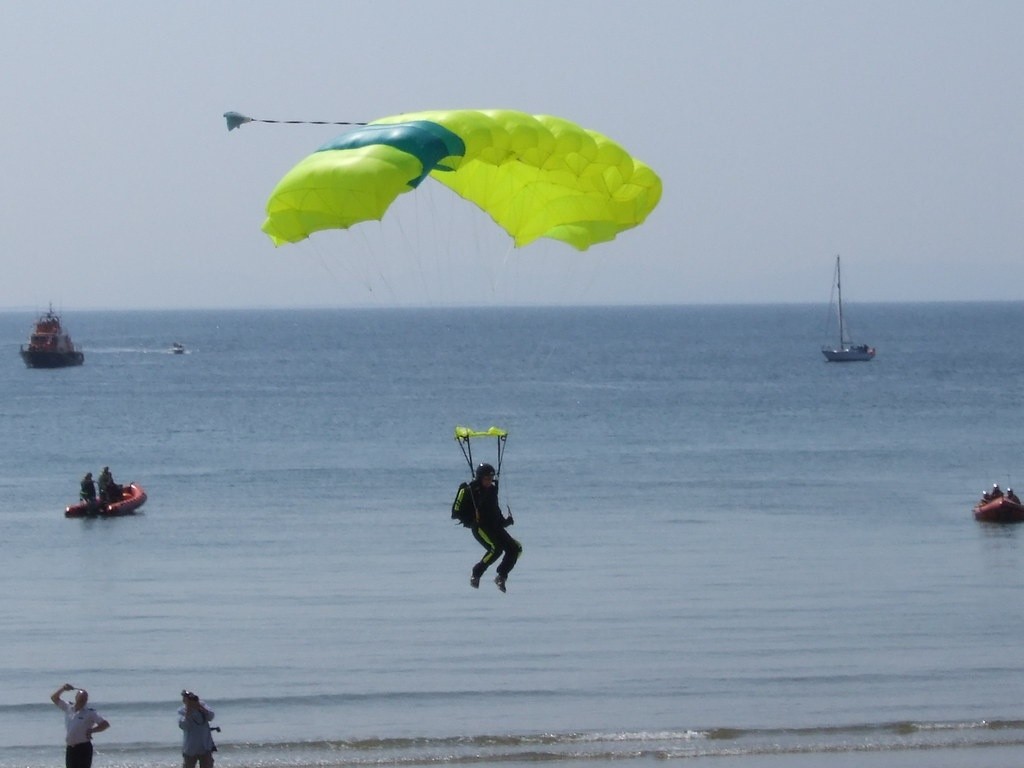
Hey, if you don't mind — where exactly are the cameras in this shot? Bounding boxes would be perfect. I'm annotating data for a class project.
[187,692,198,701]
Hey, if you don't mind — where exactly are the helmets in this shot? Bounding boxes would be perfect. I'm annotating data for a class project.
[476,463,495,480]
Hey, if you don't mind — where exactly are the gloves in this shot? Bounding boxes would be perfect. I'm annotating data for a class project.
[506,516,514,526]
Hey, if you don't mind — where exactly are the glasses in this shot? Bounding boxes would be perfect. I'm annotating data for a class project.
[484,477,494,480]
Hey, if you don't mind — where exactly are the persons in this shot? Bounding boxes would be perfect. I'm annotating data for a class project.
[51,684,109,768]
[468,463,521,593]
[982,484,1021,505]
[80,466,114,502]
[848,343,869,352]
[176,692,217,768]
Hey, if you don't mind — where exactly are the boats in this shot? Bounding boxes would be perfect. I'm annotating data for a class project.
[64,481,146,518]
[974,495,1024,522]
[21,301,85,369]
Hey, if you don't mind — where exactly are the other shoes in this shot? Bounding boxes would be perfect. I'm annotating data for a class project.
[470,572,479,588]
[494,575,506,592]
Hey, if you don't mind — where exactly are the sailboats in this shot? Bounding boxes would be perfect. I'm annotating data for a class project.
[819,251,877,362]
